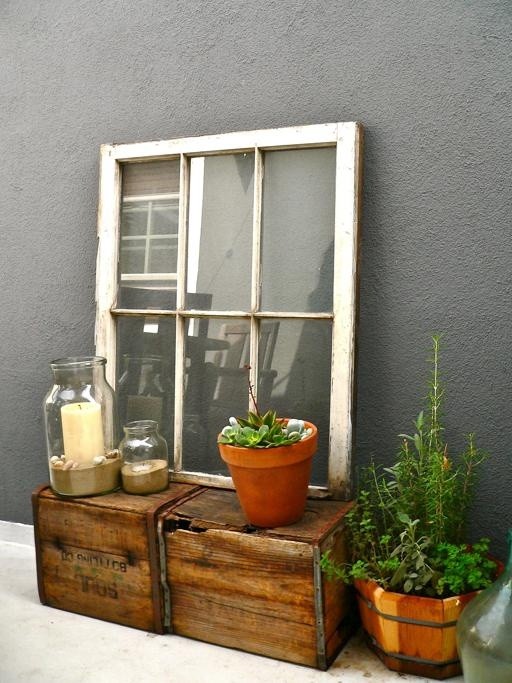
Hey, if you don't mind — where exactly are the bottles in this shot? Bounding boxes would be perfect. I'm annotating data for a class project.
[117,420,170,496]
[42,354,123,498]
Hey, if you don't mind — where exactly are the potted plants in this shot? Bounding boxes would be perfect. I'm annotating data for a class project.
[215,376,319,529]
[317,327,504,679]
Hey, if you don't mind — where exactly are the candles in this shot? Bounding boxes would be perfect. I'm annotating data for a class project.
[60,402,105,465]
